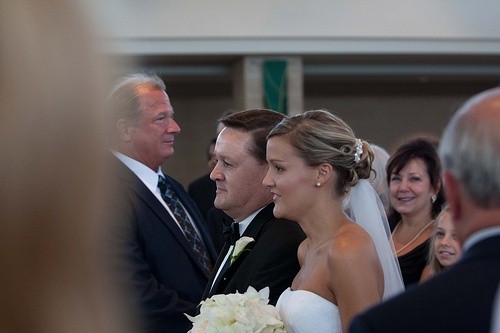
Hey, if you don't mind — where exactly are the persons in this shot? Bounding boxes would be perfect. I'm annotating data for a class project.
[0,0,500,333]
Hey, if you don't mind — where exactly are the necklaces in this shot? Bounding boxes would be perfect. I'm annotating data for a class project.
[391,219,435,253]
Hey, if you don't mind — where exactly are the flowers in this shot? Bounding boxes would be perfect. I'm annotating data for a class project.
[183,286,293,333]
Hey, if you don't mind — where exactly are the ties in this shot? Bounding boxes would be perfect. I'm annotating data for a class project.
[157,175,212,279]
